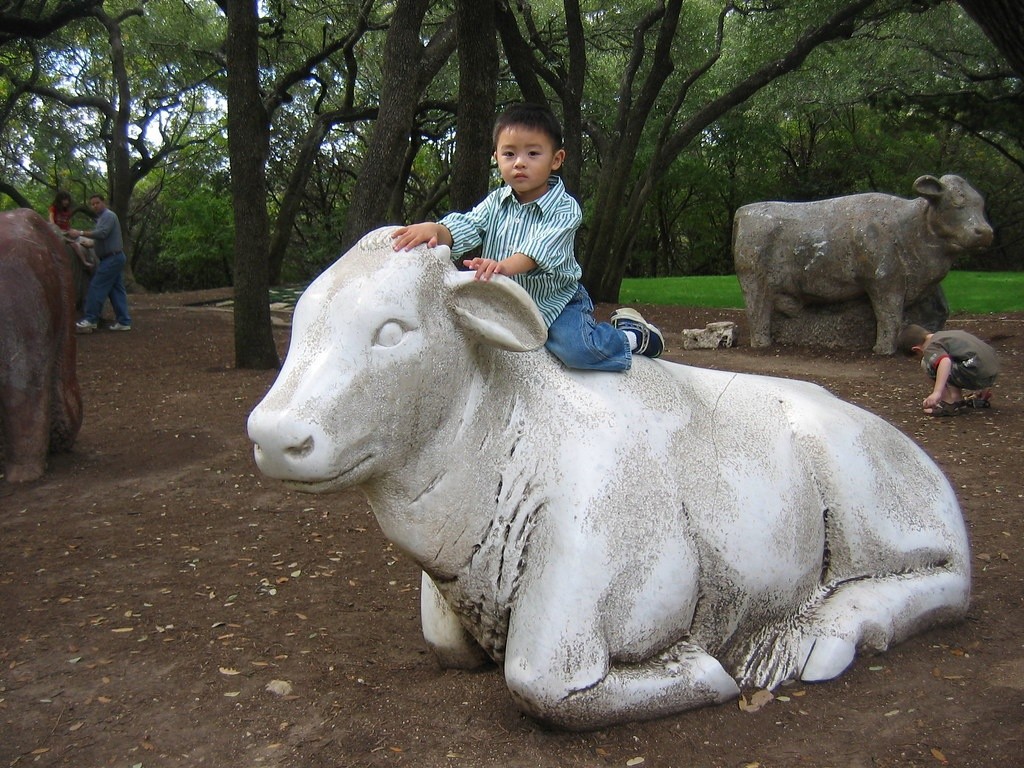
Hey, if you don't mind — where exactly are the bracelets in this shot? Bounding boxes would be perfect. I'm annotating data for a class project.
[80,230,84,236]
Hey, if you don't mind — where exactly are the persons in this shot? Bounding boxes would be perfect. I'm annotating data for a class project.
[899,324,999,417]
[67,194,132,330]
[49,191,95,267]
[391,102,666,371]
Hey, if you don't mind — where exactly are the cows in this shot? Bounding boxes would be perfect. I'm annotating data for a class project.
[0,209,101,491]
[246,224,971,731]
[733,174,994,357]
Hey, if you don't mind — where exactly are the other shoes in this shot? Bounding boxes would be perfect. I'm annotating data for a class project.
[84,262,95,269]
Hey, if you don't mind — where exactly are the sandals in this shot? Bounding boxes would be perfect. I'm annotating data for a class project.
[924,399,970,417]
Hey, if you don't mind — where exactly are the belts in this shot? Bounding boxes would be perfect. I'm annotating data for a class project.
[99,251,123,262]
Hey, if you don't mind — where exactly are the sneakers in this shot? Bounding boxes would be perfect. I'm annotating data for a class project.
[75,320,98,329]
[109,322,131,331]
[610,308,665,360]
